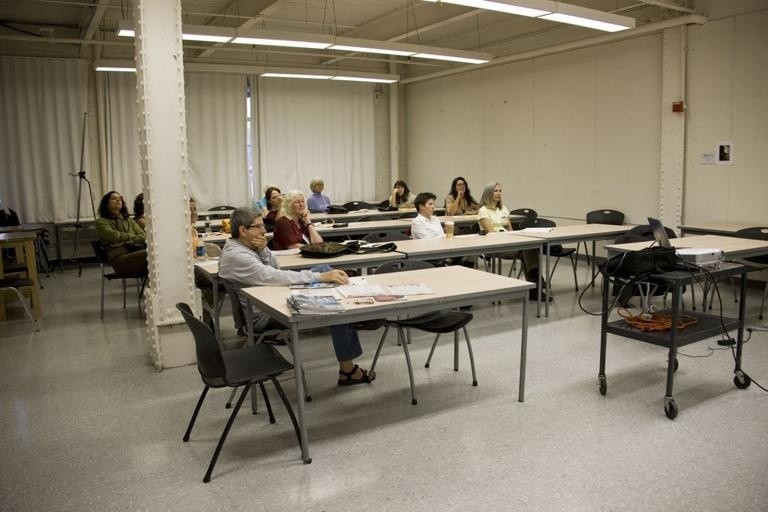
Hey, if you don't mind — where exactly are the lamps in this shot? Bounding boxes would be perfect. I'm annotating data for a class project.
[424,0,636,34]
[94,20,495,84]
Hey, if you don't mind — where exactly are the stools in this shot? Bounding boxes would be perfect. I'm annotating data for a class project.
[0,278,40,333]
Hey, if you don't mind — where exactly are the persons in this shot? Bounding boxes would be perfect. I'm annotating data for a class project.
[130,193,146,231]
[477,182,553,304]
[307,177,333,211]
[387,179,417,208]
[275,190,324,249]
[266,186,280,220]
[409,191,446,244]
[98,190,148,275]
[442,177,483,214]
[189,198,222,292]
[217,205,377,386]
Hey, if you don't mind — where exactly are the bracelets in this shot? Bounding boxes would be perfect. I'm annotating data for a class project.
[304,223,314,228]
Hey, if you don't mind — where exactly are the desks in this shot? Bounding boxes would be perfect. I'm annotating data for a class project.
[240,264,537,466]
[190,198,637,316]
[0,231,41,320]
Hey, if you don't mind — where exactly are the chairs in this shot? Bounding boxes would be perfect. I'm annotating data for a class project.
[91,240,147,319]
[174,302,304,482]
[585,207,767,320]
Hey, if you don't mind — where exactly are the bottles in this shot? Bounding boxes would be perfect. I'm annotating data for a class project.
[196,235,205,261]
[204,215,212,234]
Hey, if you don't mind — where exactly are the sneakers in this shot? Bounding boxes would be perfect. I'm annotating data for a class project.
[529,290,553,302]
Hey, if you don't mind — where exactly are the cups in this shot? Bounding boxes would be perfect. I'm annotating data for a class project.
[444,221,454,239]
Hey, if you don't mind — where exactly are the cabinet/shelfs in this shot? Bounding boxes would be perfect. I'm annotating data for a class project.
[596,258,751,421]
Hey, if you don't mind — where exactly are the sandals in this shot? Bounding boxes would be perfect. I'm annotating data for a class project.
[339,364,375,384]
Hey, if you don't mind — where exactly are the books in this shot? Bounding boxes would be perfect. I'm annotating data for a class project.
[286,293,347,318]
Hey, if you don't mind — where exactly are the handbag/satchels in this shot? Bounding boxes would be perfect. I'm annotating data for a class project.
[299,242,360,258]
[597,246,676,276]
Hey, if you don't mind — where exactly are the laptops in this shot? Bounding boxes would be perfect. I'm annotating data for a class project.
[647,216,697,253]
